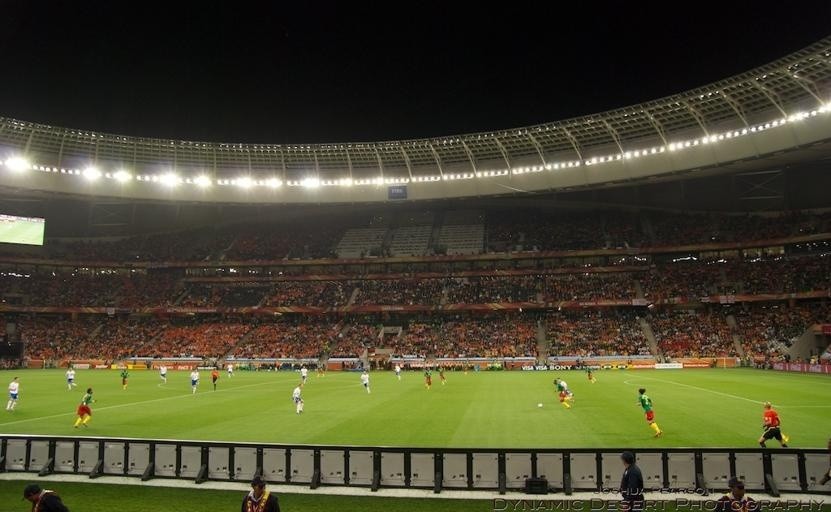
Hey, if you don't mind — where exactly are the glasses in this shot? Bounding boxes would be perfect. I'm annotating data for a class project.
[258,484,266,489]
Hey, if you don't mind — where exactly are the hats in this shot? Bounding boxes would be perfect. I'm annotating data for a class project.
[251,476,267,485]
[20,485,41,502]
[727,476,746,487]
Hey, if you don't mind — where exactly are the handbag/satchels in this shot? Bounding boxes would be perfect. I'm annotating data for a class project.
[525,477,548,495]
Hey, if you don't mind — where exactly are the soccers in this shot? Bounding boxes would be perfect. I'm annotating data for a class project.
[537,403,544,408]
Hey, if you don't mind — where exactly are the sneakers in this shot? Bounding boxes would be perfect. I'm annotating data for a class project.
[82,423,89,428]
[654,431,662,438]
[73,426,79,429]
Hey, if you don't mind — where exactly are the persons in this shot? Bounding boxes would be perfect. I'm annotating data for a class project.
[368,359,393,371]
[208,367,221,392]
[300,365,308,384]
[0,359,19,370]
[552,376,575,409]
[626,355,718,368]
[225,362,233,380]
[309,359,328,377]
[585,364,597,384]
[713,478,762,512]
[204,359,217,367]
[258,361,302,371]
[360,370,370,395]
[619,450,644,512]
[65,366,78,391]
[436,361,475,371]
[394,363,402,381]
[73,387,97,430]
[1,198,830,360]
[399,360,433,371]
[557,378,574,398]
[341,360,360,370]
[437,364,447,386]
[159,363,168,385]
[235,362,257,371]
[23,484,69,512]
[241,477,280,512]
[292,382,304,416]
[189,368,200,395]
[424,367,432,390]
[120,368,129,390]
[637,386,663,438]
[740,356,831,370]
[5,376,20,411]
[757,401,789,448]
